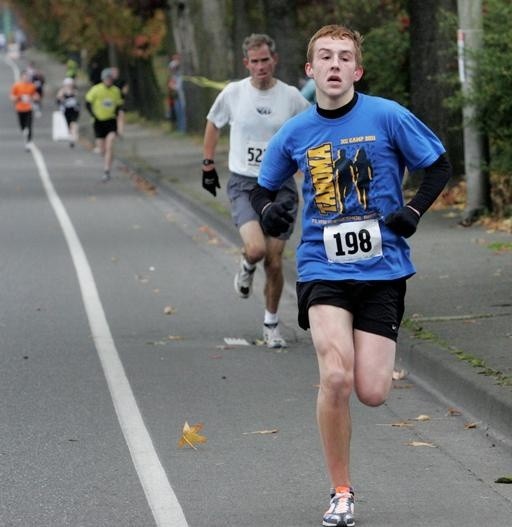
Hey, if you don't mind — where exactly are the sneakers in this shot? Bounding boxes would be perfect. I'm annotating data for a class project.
[322,484,357,526]
[261,321,288,349]
[233,255,257,299]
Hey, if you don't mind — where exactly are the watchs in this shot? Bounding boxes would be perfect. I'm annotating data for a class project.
[202,158,214,165]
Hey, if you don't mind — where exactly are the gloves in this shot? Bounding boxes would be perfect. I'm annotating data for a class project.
[259,196,295,240]
[200,169,221,198]
[384,205,420,238]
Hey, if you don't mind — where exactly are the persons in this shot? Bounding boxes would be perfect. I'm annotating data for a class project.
[10,56,129,180]
[202,32,317,348]
[250,26,453,527]
[167,61,187,138]
